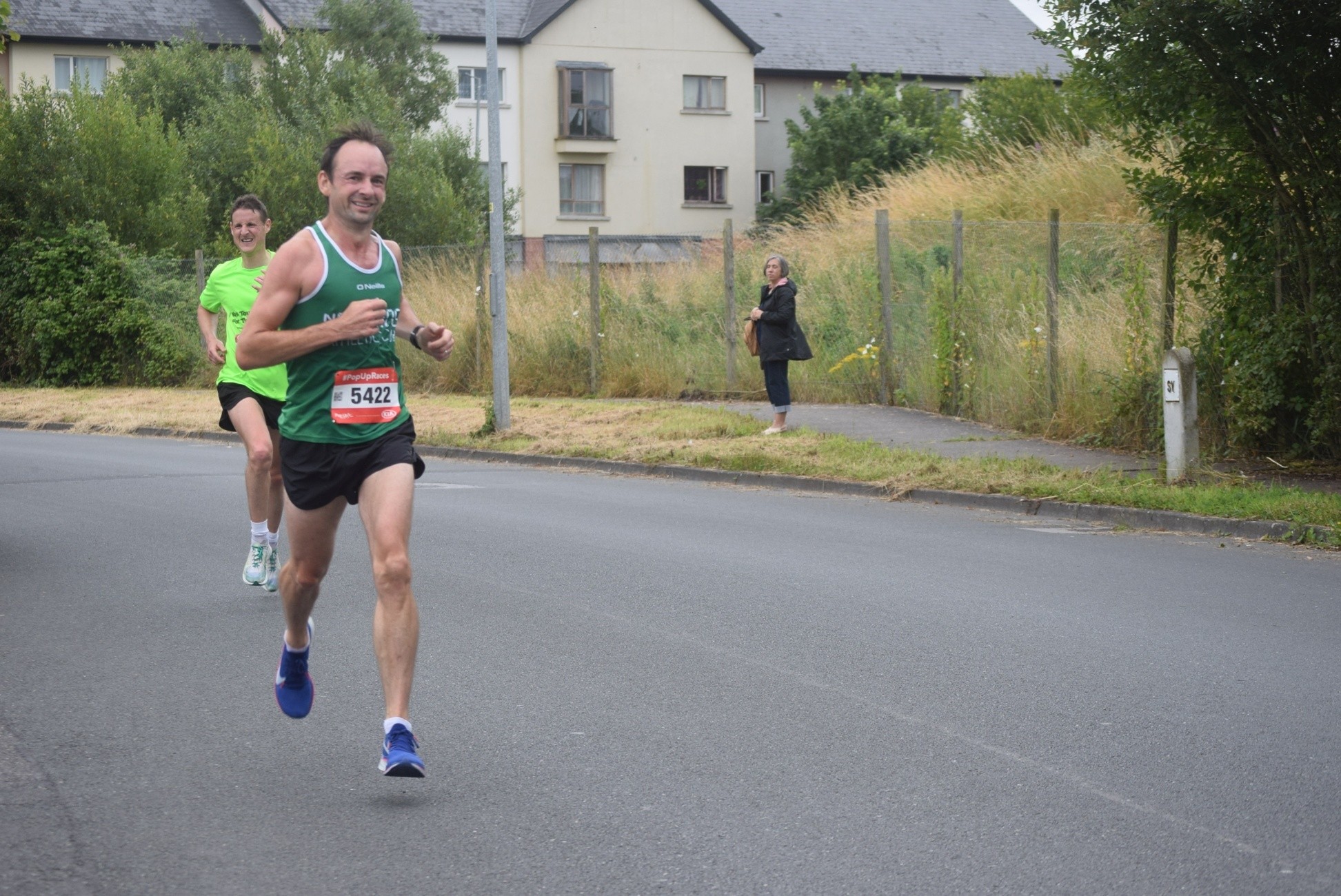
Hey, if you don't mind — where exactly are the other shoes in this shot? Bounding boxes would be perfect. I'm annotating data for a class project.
[759,425,787,435]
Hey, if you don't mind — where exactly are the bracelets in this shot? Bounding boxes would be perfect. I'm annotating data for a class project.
[410,325,425,350]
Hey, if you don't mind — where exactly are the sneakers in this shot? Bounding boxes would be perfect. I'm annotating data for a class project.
[274,615,315,718]
[378,723,426,778]
[242,537,273,586]
[261,541,282,592]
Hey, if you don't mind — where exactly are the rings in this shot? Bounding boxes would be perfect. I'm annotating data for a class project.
[445,350,449,356]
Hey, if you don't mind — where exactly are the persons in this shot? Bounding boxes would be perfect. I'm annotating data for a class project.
[750,256,813,435]
[198,195,290,592]
[235,121,456,778]
[570,100,605,137]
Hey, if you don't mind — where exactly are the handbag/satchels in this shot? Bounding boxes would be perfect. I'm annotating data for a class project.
[743,320,760,356]
